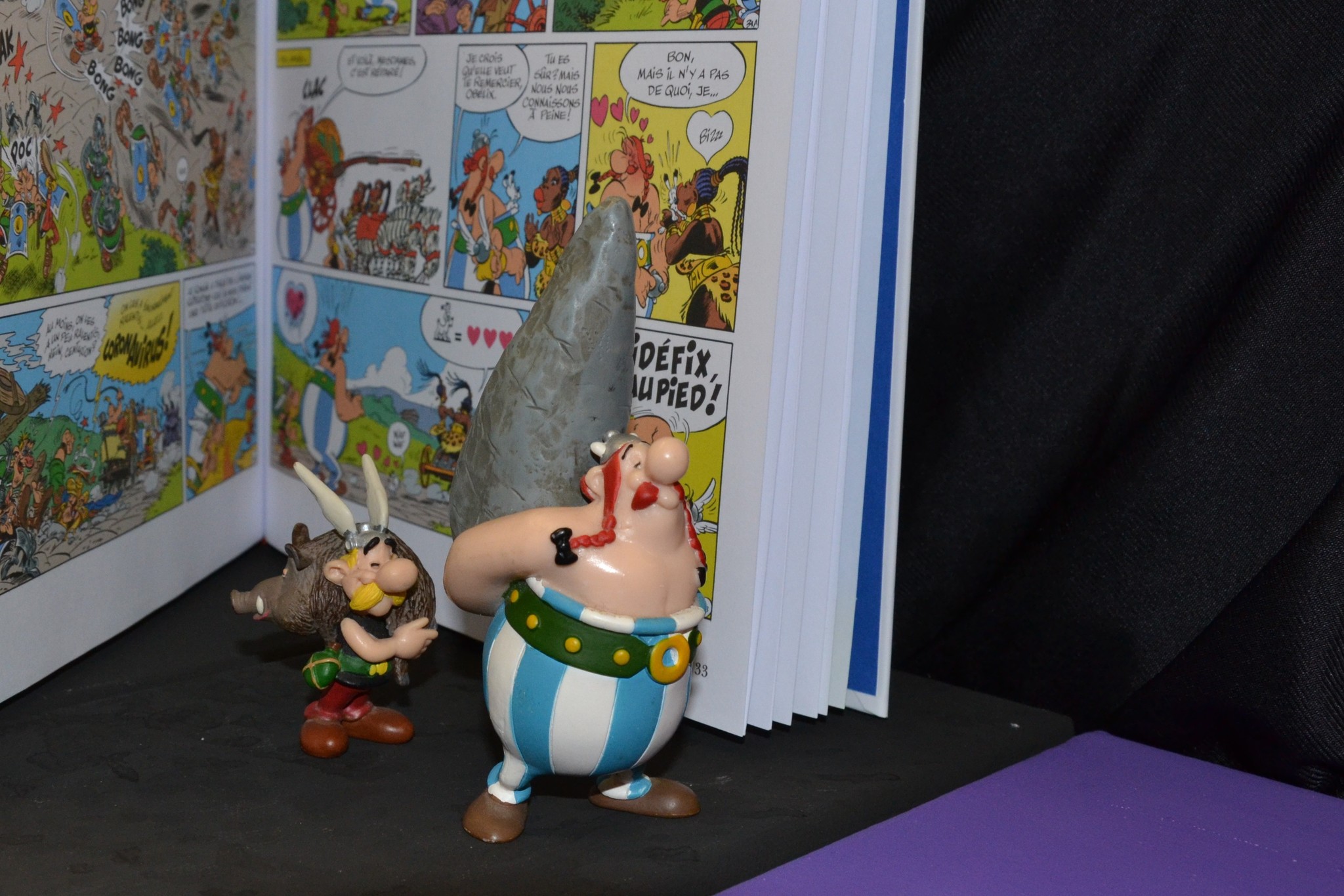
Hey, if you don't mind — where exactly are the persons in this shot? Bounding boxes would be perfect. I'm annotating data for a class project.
[292,453,436,759]
[445,430,708,841]
